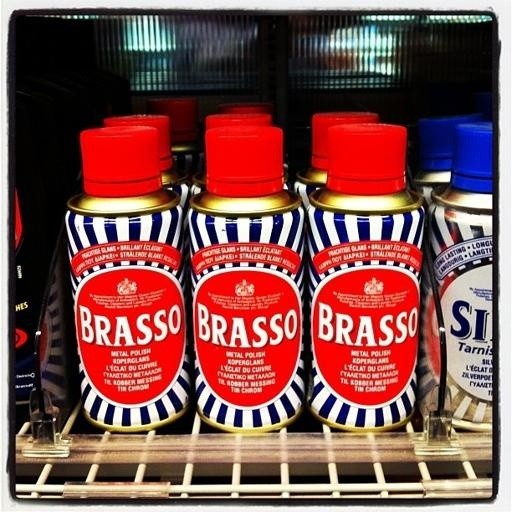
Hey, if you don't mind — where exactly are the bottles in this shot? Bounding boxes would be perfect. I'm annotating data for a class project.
[62,95,191,434]
[295,108,428,435]
[413,119,493,434]
[189,108,306,436]
